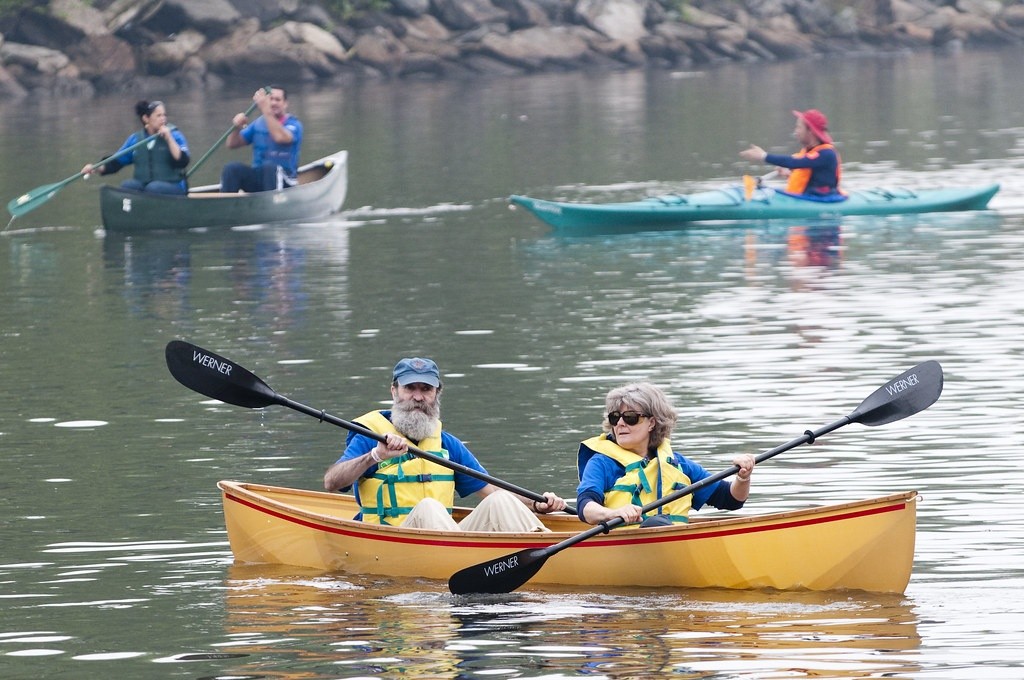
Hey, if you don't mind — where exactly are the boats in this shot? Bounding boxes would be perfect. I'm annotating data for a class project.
[98,149,350,232]
[503,180,1003,239]
[216,477,927,598]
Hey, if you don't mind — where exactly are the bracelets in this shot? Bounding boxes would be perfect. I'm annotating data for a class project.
[371,447,383,463]
[533,501,547,515]
[761,152,768,163]
[737,474,751,482]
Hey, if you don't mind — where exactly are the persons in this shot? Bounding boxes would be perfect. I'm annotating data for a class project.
[80,98,192,198]
[737,110,846,201]
[219,84,304,190]
[574,385,754,532]
[323,356,569,533]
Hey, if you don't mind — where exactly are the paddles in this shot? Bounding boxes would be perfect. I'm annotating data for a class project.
[7,127,181,218]
[183,85,273,180]
[449,358,946,595]
[162,338,580,517]
[741,155,797,200]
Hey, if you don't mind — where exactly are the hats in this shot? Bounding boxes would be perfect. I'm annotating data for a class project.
[792,108,833,143]
[393,356,443,388]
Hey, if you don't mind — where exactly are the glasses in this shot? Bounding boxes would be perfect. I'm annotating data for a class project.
[606,410,652,427]
[147,100,166,110]
